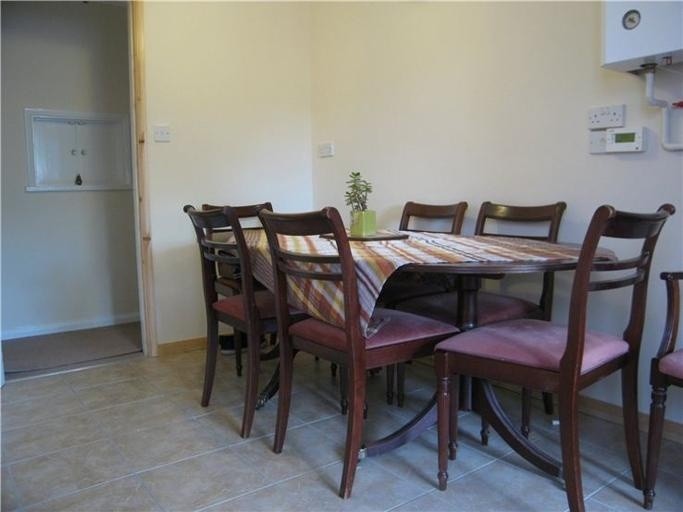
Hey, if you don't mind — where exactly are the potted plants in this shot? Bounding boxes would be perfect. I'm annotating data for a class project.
[344,172,377,237]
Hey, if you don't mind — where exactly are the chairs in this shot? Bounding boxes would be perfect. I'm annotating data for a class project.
[259,207,462,499]
[433,202,678,510]
[587,104,624,130]
[182,200,568,479]
[644,271,681,511]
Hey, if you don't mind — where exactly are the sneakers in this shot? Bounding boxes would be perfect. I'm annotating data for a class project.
[219,336,267,355]
